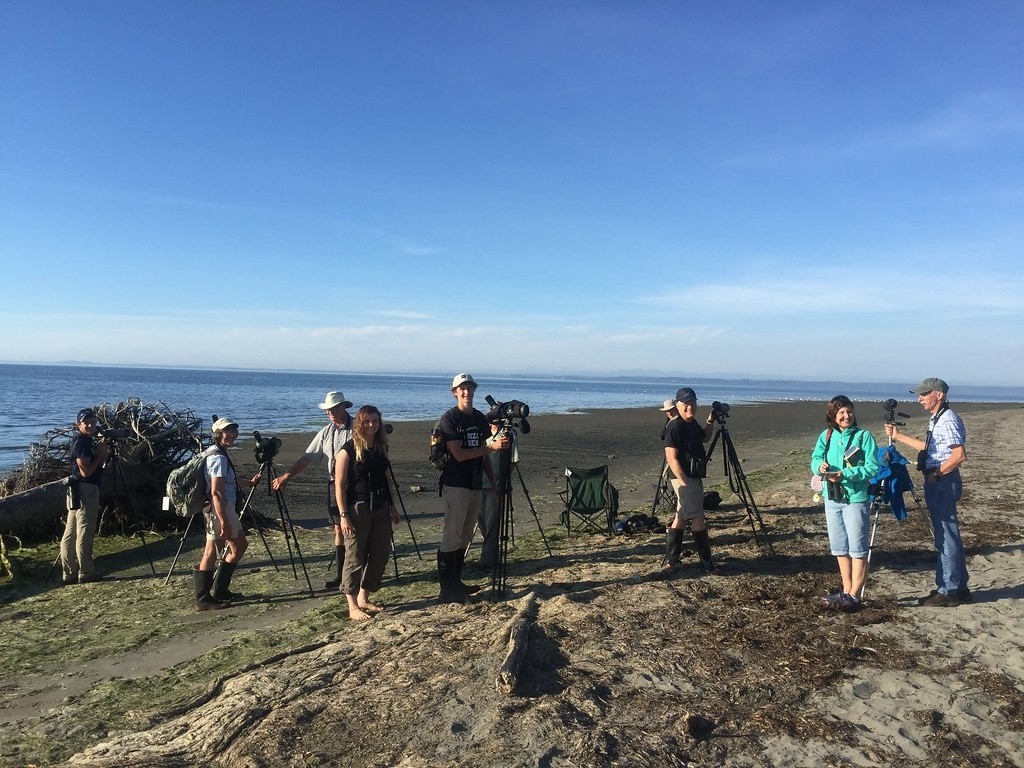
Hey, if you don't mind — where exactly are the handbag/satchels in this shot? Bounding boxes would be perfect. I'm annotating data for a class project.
[810,428,834,496]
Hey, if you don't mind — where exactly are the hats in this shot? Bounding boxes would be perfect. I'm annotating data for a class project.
[908,377,949,394]
[76,408,96,422]
[659,399,676,412]
[450,372,479,391]
[676,387,698,403]
[317,390,353,410]
[211,417,240,433]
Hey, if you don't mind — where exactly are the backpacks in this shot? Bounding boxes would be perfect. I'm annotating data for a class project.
[429,408,482,473]
[164,449,230,518]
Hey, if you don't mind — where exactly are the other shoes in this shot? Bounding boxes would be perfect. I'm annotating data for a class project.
[63,577,78,585]
[820,590,866,614]
[77,577,100,584]
[917,590,973,607]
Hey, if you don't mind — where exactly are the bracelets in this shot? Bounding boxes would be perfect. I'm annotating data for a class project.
[341,512,348,517]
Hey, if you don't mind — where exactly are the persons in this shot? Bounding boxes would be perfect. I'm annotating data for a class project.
[60,408,108,584]
[659,400,707,524]
[884,378,967,607]
[273,391,356,588]
[336,405,399,620]
[437,372,510,603]
[468,402,519,571]
[811,396,879,609]
[193,418,262,611]
[665,388,728,574]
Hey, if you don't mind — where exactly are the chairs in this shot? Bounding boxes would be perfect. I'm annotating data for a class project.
[557,464,621,538]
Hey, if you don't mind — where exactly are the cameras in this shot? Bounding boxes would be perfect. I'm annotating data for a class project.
[712,401,729,418]
[101,429,131,438]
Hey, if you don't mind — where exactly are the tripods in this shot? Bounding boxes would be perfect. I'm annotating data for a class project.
[213,456,315,598]
[489,418,553,604]
[328,454,423,582]
[163,483,279,587]
[660,415,786,580]
[45,437,158,583]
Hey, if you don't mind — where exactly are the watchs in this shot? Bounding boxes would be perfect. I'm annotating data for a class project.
[933,471,939,479]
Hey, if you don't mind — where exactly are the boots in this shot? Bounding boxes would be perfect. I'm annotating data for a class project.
[436,548,481,605]
[192,565,232,612]
[211,558,243,601]
[665,528,691,573]
[692,527,725,576]
[323,544,345,589]
